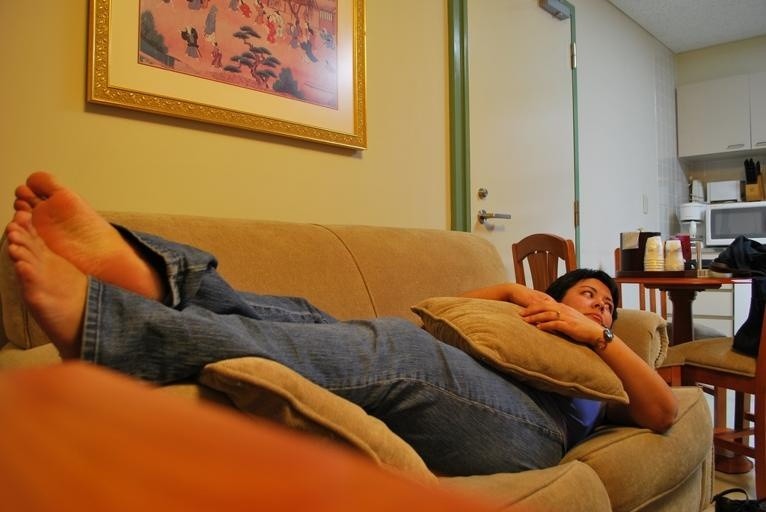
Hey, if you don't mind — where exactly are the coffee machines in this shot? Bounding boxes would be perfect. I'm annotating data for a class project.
[678,202,705,253]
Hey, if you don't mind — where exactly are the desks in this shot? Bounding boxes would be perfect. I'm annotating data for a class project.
[607,275,736,343]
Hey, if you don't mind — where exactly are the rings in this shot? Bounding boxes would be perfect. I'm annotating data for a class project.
[555,308,562,319]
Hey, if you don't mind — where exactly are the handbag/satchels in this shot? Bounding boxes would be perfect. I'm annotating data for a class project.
[712,487,760,512]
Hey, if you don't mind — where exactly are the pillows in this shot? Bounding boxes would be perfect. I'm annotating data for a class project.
[198,354,443,488]
[411,291,633,405]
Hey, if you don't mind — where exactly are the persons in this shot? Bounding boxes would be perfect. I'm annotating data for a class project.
[211,41,223,69]
[186,0,210,13]
[5,170,678,474]
[178,23,203,58]
[228,0,336,76]
[203,5,219,41]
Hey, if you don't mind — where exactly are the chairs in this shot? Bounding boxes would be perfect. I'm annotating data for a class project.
[612,247,695,346]
[511,232,689,392]
[682,290,766,505]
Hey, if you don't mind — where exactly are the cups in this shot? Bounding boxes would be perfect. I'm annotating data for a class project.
[674,234,691,262]
[644,236,665,271]
[665,240,687,273]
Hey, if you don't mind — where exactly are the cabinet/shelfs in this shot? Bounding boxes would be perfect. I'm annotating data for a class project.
[675,71,766,163]
[621,282,765,438]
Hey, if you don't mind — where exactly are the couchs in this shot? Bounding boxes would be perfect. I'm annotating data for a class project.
[1,212,715,511]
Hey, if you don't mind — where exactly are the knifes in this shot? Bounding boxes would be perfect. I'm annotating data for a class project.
[744,157,762,184]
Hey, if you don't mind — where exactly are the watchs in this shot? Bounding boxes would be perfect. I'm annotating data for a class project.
[590,324,615,351]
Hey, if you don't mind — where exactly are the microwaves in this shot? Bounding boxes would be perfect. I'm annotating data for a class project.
[705,200,766,246]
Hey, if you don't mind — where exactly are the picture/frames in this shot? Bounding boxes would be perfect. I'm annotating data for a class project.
[82,0,368,158]
